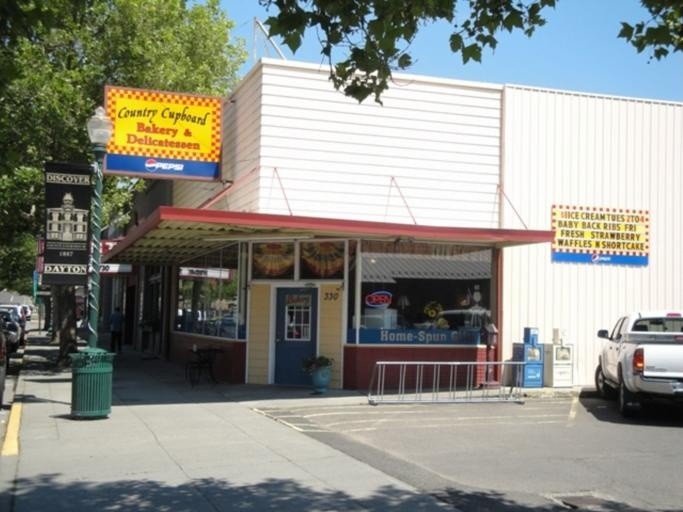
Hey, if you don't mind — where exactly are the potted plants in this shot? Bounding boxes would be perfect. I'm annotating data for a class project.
[304,357,334,397]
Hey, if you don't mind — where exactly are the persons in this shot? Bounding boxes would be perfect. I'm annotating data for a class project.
[108,306,126,354]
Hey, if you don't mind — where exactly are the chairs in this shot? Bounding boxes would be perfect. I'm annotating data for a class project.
[186,345,225,390]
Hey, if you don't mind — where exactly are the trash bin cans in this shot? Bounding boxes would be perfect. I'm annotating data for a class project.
[69,352,117,419]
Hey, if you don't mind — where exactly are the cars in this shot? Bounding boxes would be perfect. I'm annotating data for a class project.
[593,311,681,417]
[0,304,32,409]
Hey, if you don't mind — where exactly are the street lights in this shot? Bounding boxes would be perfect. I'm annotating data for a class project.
[86,104,113,347]
